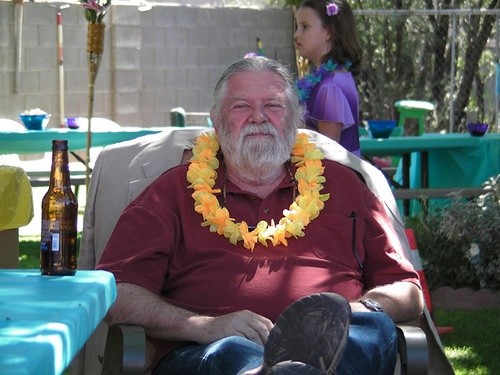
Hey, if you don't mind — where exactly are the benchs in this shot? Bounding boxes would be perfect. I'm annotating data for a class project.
[23,168,85,186]
[390,189,481,199]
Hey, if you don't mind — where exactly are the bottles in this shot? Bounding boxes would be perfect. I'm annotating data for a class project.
[40,140,78,276]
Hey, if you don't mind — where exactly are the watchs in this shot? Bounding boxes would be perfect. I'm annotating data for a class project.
[357,298,383,312]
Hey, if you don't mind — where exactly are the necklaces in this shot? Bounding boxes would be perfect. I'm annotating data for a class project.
[187,128,329,252]
[294,58,352,100]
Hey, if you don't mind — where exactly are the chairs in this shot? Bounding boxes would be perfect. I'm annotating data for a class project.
[106,146,426,375]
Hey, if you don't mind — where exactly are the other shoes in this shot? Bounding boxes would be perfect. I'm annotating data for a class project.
[240,292,352,375]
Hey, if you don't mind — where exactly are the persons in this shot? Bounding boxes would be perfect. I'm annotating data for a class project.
[294,0,362,159]
[93,55,424,375]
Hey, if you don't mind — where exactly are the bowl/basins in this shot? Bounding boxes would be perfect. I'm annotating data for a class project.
[19,113,52,131]
[67,117,81,128]
[467,124,489,137]
[362,120,398,139]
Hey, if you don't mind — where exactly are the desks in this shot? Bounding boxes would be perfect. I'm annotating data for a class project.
[359,133,500,218]
[0,127,164,201]
[0,269,118,374]
